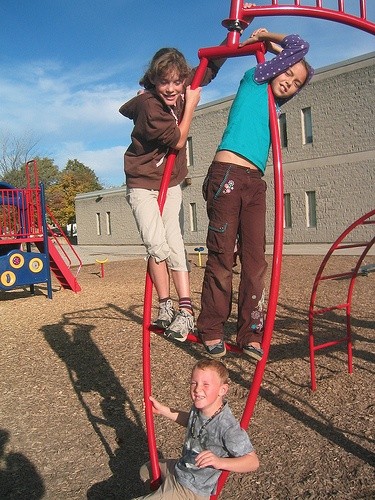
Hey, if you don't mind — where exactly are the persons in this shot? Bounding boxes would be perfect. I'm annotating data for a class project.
[196,28,314,361]
[133,359,259,500]
[119,2,255,342]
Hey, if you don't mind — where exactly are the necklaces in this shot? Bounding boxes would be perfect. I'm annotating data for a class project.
[191,404,224,439]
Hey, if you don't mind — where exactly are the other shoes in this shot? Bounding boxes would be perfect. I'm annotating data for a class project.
[242,343,264,361]
[202,339,226,358]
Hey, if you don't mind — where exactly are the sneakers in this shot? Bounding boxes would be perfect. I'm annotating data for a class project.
[157,299,175,329]
[163,309,195,343]
